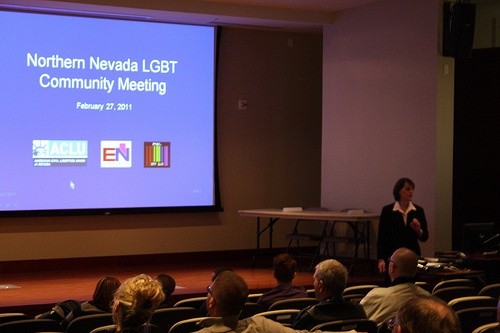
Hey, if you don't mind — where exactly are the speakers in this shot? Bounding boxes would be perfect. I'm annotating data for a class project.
[449,2,476,49]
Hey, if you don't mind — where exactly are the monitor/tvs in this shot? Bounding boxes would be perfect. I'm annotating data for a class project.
[463,222,496,250]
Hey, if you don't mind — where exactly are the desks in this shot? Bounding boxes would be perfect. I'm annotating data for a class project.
[237,208,381,276]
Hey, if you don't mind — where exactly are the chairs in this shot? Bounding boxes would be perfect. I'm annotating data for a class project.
[321,208,368,276]
[285,206,330,273]
[0,278,500,333]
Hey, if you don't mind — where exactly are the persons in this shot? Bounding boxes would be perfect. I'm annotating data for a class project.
[60,247,463,333]
[377,178,429,280]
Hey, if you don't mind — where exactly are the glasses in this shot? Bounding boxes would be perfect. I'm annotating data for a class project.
[207,284,217,301]
[388,257,394,263]
